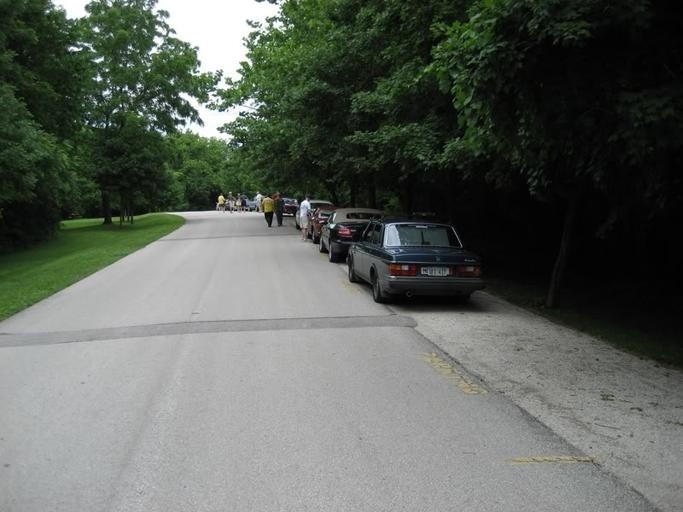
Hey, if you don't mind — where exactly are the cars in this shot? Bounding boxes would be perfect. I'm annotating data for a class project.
[216,197,299,217]
[295,199,385,262]
[344,215,489,303]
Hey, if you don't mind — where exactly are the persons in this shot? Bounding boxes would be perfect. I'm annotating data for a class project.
[299,196,313,241]
[218,191,265,214]
[260,192,287,228]
